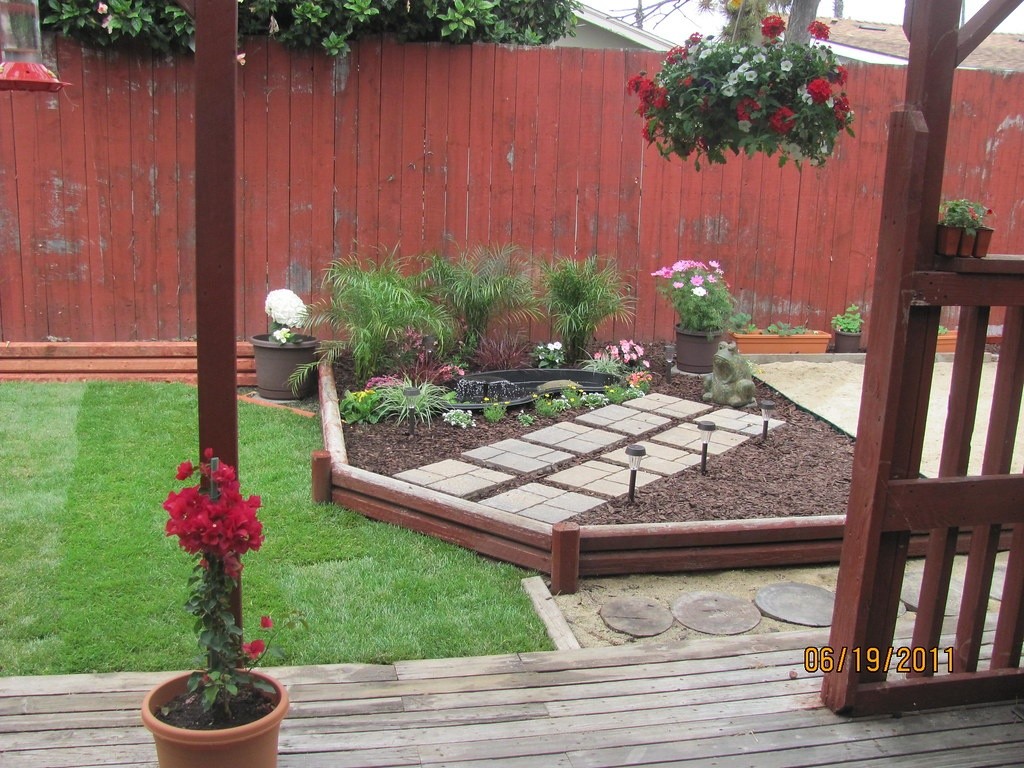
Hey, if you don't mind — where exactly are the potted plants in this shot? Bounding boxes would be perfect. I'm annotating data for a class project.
[831,304,865,353]
[935,325,960,353]
[727,313,832,354]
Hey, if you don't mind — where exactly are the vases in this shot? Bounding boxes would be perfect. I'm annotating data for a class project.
[250,334,318,401]
[957,227,976,258]
[938,223,962,256]
[973,226,994,259]
[674,323,724,374]
[142,670,289,768]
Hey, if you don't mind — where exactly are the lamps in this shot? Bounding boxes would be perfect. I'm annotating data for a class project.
[760,399,775,422]
[0,0,75,94]
[697,421,717,444]
[626,445,647,470]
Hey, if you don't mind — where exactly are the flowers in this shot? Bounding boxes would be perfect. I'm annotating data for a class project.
[626,15,858,171]
[650,260,738,342]
[264,288,309,347]
[938,199,997,236]
[162,448,277,723]
